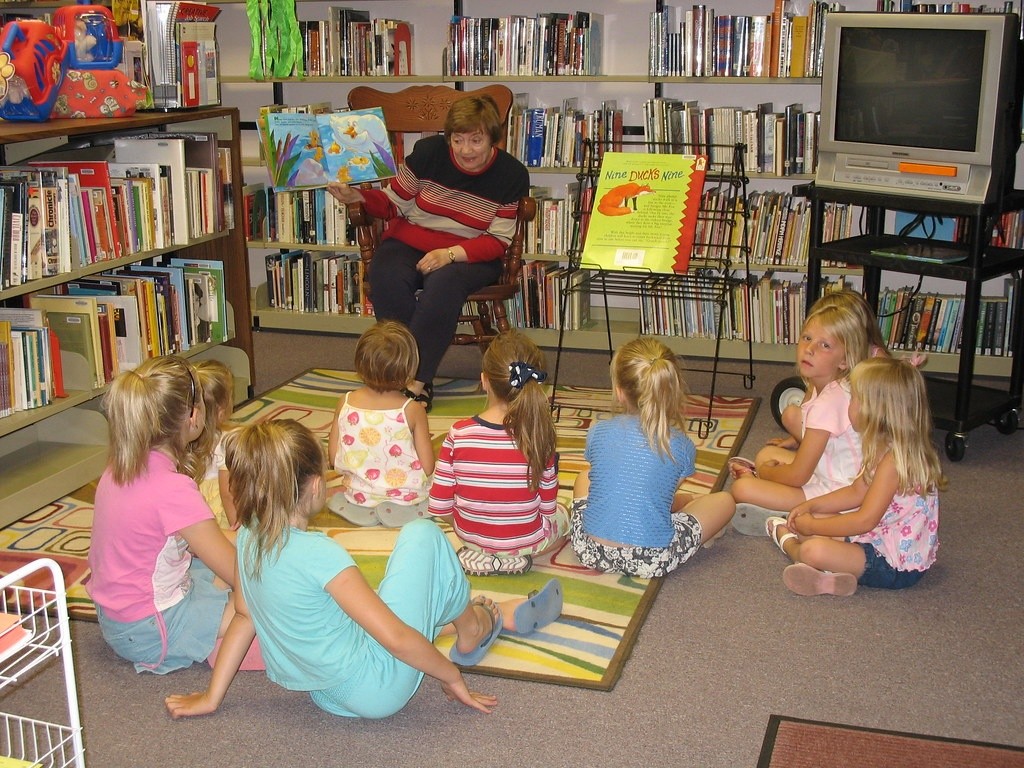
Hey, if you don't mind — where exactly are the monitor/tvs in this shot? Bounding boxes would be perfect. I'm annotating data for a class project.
[815,12,1024,205]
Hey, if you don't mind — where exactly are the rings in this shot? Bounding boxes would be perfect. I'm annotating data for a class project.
[428,267,432,271]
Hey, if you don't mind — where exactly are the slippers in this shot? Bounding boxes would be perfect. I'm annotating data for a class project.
[448,597,502,667]
[511,579,564,634]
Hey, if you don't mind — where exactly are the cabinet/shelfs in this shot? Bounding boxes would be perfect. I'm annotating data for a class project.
[547,138,755,442]
[770,178,1024,463]
[0,557,87,768]
[206,0,1024,380]
[0,107,256,531]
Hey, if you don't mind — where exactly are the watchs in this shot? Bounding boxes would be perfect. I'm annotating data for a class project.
[448,249,455,263]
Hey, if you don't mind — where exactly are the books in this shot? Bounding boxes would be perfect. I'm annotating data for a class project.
[446,10,601,76]
[292,7,411,76]
[638,0,1024,357]
[234,103,623,330]
[0,132,235,420]
[255,106,398,193]
[162,0,220,84]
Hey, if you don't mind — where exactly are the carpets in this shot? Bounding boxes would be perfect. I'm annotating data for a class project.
[0,366,761,693]
[758,714,1024,768]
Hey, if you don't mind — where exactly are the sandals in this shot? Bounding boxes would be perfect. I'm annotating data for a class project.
[783,563,857,597]
[728,457,756,479]
[401,388,417,400]
[414,382,433,413]
[731,503,791,536]
[765,517,800,554]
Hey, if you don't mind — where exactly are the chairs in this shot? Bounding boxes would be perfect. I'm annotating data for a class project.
[345,84,539,355]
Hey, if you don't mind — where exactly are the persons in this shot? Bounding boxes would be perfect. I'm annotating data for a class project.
[809,290,891,358]
[327,322,435,527]
[165,419,563,718]
[83,354,266,674]
[571,337,736,577]
[728,305,868,538]
[328,96,530,414]
[191,359,237,528]
[427,331,572,576]
[766,357,948,597]
